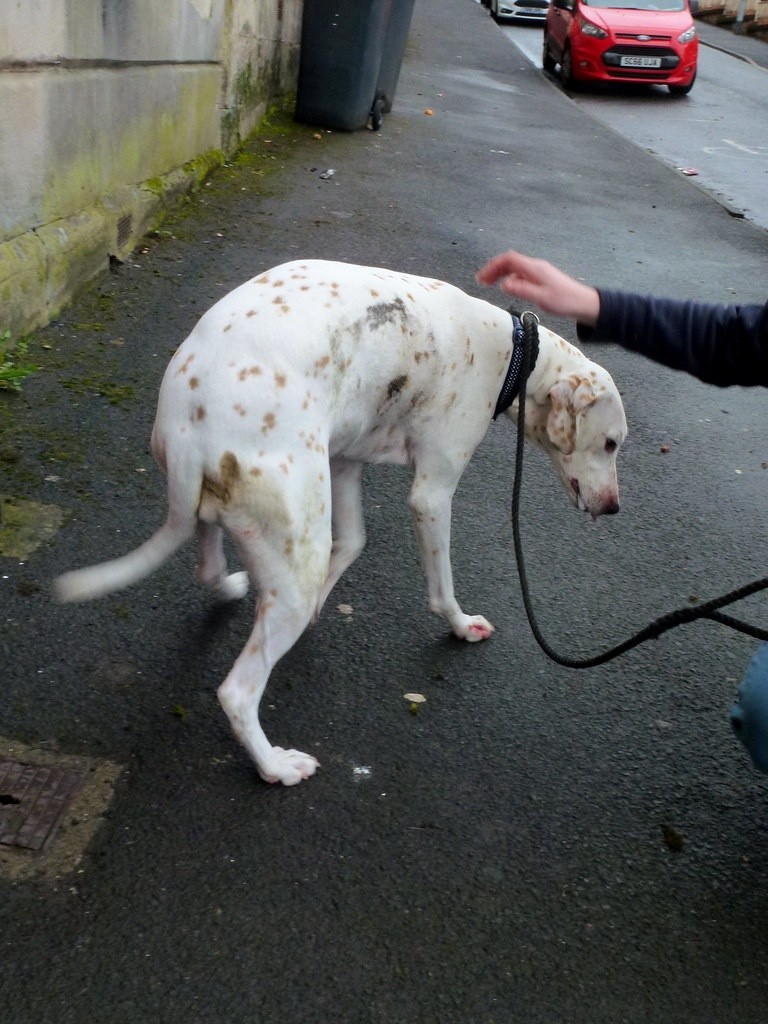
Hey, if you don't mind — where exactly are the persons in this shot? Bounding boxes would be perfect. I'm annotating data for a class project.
[473,249,767,771]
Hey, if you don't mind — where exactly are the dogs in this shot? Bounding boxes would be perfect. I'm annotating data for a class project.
[52,256,628,786]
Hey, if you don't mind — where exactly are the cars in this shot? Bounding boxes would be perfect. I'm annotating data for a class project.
[542,0,699,95]
[480,0,551,25]
